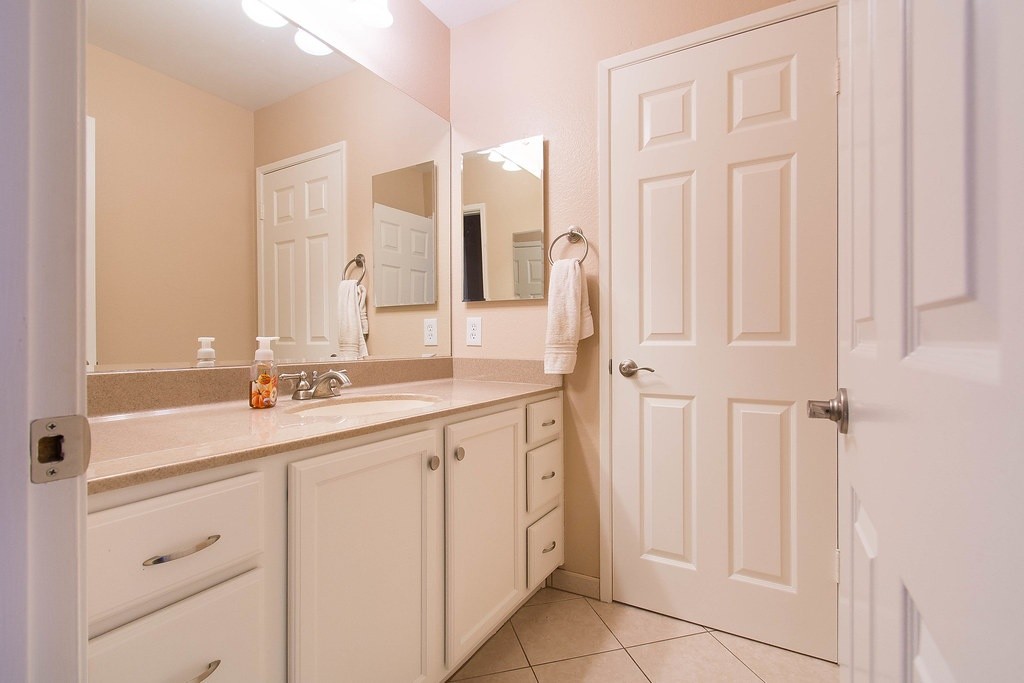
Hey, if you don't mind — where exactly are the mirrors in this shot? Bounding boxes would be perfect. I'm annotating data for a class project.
[374,161,436,308]
[85,1,450,374]
[461,134,544,304]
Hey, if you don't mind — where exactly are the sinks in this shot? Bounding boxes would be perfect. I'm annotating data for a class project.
[282,394,441,417]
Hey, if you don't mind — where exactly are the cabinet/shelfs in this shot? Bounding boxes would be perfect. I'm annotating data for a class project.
[86,392,564,683]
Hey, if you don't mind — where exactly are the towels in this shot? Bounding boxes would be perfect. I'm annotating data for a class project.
[544,258,594,375]
[338,280,370,357]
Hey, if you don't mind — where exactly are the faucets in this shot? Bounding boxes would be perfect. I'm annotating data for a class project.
[278,369,352,400]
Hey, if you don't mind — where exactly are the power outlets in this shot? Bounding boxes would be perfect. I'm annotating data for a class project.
[424,319,437,345]
[466,317,482,347]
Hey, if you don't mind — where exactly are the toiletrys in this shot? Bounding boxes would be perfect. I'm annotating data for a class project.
[189,336,219,367]
[248,336,281,408]
